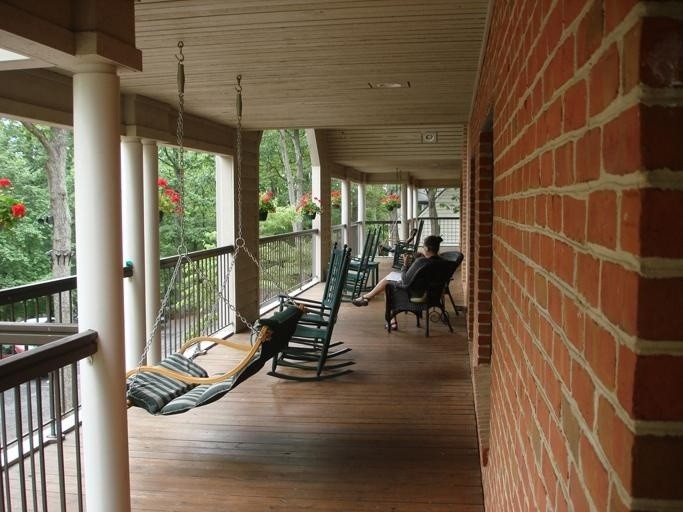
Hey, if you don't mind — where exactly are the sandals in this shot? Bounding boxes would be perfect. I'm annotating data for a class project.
[352,297,368,306]
[385,321,397,330]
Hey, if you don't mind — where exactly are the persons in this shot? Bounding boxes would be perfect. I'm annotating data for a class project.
[352,235,443,330]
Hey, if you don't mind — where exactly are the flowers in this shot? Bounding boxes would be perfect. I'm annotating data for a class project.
[295,190,341,216]
[382,192,399,208]
[156,179,179,216]
[257,191,276,213]
[0,176,26,230]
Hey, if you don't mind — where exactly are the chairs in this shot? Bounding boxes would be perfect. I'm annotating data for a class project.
[390,219,424,271]
[381,250,464,338]
[266,226,381,382]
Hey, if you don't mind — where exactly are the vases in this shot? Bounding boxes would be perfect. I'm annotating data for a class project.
[303,212,315,220]
[386,205,393,211]
[258,209,268,222]
